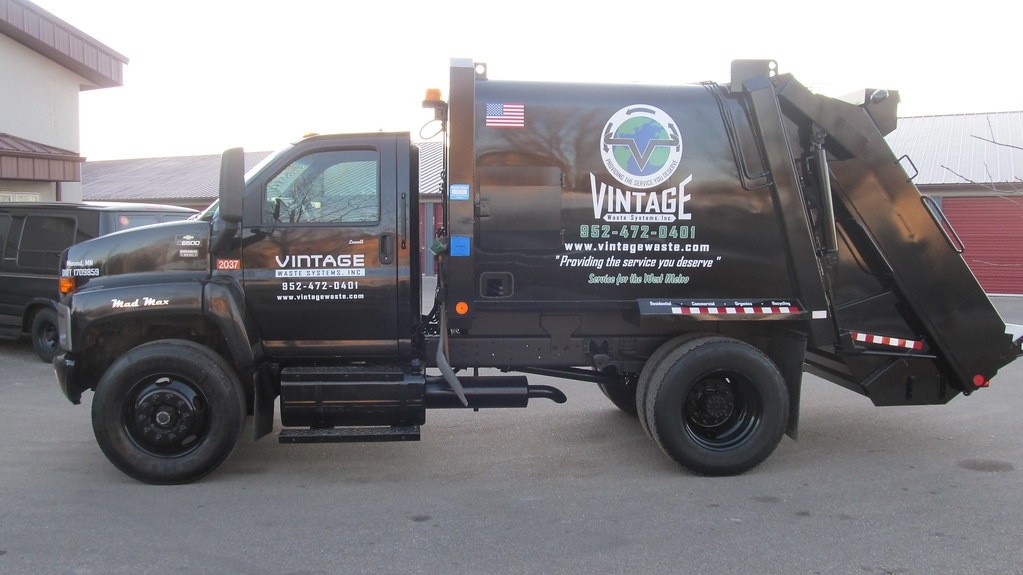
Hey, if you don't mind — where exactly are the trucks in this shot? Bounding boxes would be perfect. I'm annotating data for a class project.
[51,57,1023,485]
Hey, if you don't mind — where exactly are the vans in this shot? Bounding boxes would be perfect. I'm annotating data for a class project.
[1,203,203,364]
